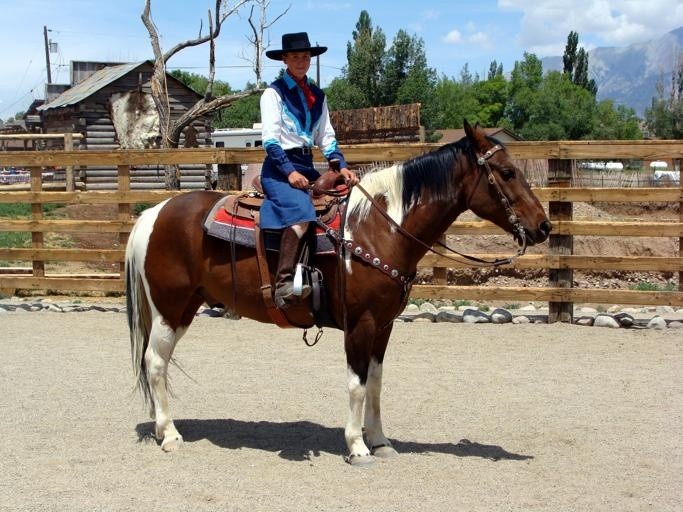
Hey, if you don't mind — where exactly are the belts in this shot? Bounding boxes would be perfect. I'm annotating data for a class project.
[284,146,312,155]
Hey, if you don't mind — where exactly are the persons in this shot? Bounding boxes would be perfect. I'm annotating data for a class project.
[258,31,361,309]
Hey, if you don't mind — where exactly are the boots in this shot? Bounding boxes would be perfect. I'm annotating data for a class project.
[276,227,312,310]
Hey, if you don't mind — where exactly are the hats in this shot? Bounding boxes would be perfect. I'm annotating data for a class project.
[266,33,327,60]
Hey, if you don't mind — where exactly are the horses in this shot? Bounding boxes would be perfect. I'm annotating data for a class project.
[124,117,553,466]
